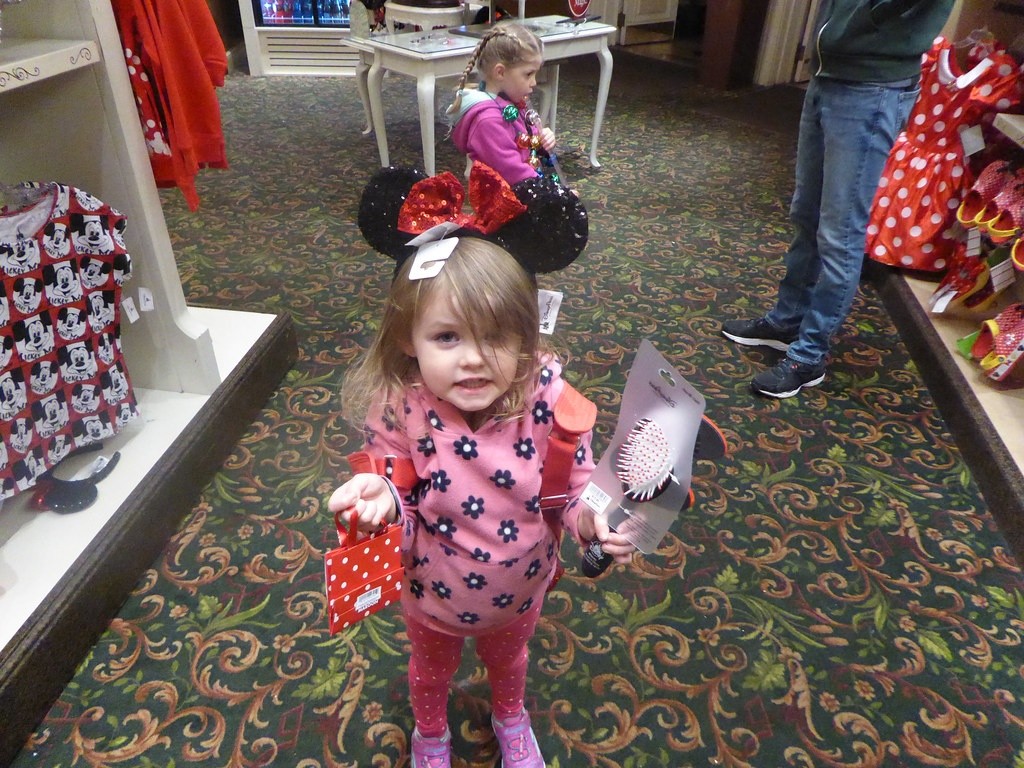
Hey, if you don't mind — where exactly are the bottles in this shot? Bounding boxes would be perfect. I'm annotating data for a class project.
[261,0,350,24]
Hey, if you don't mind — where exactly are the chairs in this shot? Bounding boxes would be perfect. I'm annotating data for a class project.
[348,0,371,36]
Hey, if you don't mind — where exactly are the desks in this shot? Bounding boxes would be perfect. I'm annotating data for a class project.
[377,0,489,36]
[339,12,619,184]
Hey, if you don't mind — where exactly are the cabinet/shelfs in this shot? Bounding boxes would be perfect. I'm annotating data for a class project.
[0,0,303,768]
[893,0,1024,518]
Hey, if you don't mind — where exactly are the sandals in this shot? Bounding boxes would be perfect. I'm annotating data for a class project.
[971,302,1024,362]
[988,195,1024,248]
[1011,234,1024,272]
[980,321,1024,382]
[932,244,1012,311]
[975,171,1024,233]
[956,160,1014,232]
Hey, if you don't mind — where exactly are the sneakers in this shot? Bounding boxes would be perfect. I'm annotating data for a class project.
[751,356,826,399]
[721,317,797,351]
[491,706,547,768]
[411,723,452,768]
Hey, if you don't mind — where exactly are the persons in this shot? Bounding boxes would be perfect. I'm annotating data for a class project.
[721,0,962,398]
[328,165,645,768]
[444,19,557,186]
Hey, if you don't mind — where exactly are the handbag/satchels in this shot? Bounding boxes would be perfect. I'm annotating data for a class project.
[325,505,406,636]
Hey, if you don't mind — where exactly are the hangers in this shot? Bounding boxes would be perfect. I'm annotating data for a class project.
[942,12,1002,62]
[0,173,64,230]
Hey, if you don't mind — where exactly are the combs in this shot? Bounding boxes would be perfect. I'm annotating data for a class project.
[581,411,684,579]
